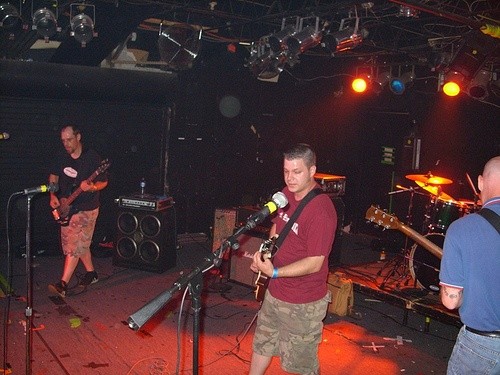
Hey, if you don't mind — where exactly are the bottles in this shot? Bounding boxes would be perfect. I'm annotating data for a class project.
[139,177,146,195]
[379,247,386,261]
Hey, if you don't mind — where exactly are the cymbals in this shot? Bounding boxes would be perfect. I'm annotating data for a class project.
[405,175,455,201]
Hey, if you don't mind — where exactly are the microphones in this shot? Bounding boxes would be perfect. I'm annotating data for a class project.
[15,182,59,195]
[0,132,10,139]
[246,191,288,230]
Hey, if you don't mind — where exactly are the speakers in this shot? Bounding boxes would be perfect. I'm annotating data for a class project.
[112,205,177,273]
[210,208,277,287]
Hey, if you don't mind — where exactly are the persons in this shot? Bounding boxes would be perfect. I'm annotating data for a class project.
[251,142,338,375]
[49,123,109,298]
[439,155,500,375]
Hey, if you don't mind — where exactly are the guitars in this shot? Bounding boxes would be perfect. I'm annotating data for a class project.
[364,204,443,259]
[252,236,277,302]
[51,158,112,227]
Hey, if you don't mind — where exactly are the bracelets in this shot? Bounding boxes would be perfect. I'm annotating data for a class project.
[272,267,278,279]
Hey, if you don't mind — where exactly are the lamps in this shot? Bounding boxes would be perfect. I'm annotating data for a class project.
[0,0,500,100]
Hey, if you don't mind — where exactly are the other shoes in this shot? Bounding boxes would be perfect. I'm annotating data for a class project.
[48,282,65,299]
[79,271,98,286]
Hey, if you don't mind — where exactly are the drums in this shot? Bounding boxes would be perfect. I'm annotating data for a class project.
[407,199,480,297]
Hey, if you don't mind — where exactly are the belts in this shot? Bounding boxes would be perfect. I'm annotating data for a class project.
[464,325,500,338]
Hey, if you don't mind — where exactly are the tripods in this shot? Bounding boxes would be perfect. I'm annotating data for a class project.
[378,182,429,290]
